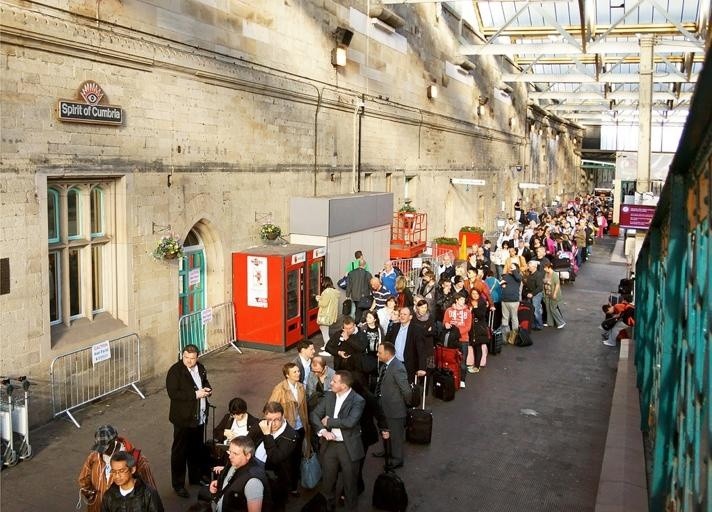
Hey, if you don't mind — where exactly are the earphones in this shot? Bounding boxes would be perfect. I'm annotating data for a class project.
[114,441,118,445]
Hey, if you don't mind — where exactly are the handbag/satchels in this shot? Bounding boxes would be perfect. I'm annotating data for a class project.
[514,328,533,347]
[465,343,488,367]
[618,278,633,294]
[551,251,579,282]
[300,452,322,489]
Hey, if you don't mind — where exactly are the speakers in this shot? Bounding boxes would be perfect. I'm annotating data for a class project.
[336,28,353,47]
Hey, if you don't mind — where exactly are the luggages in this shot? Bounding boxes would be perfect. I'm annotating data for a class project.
[203,404,229,482]
[487,310,502,354]
[406,373,432,444]
[518,296,536,332]
[433,326,461,401]
[372,430,409,512]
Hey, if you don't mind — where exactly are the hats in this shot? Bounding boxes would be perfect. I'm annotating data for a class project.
[90,425,117,453]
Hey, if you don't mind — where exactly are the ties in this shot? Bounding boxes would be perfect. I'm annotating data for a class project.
[376,364,386,400]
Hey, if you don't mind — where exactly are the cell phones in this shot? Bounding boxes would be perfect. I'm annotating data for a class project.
[82,487,96,492]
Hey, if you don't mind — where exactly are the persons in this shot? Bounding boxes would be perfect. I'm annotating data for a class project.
[79,423,159,511]
[436,240,502,388]
[345,250,440,406]
[573,193,613,254]
[167,343,213,497]
[494,219,566,342]
[98,452,164,511]
[514,198,586,285]
[316,276,341,356]
[603,296,635,346]
[208,311,413,511]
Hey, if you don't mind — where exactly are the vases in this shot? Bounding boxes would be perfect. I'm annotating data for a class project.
[266,232,277,241]
[163,251,178,259]
[459,232,483,247]
[401,215,414,229]
[431,243,459,261]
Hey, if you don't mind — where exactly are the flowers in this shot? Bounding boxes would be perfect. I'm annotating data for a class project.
[434,236,459,245]
[460,225,485,234]
[151,236,185,260]
[399,206,416,216]
[258,223,281,240]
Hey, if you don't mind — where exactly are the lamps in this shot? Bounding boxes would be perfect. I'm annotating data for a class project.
[427,85,437,97]
[331,47,347,67]
[478,95,489,105]
